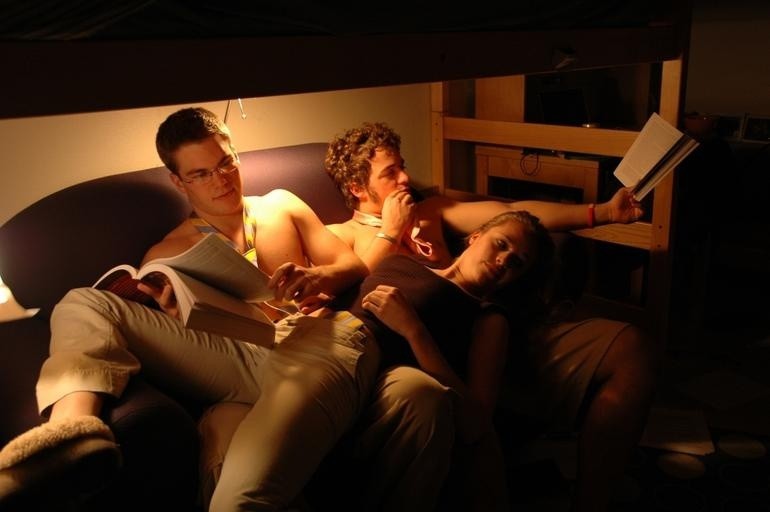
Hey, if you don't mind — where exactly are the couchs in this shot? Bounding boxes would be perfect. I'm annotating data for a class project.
[0,142,575,510]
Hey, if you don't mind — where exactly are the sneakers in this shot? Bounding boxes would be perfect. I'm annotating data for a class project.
[0,416,123,499]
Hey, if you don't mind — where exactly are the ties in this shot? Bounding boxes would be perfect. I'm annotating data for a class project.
[189,202,364,330]
[352,209,444,263]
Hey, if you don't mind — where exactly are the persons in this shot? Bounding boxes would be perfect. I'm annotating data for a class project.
[323,120,655,510]
[1,210,552,511]
[140,106,465,511]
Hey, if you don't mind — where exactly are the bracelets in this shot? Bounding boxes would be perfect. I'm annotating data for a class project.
[379,233,399,246]
[587,203,597,229]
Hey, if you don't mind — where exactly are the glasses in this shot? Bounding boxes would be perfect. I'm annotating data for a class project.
[180,151,239,185]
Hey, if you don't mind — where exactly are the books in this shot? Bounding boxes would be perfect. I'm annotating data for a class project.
[613,111,701,202]
[92,232,278,349]
